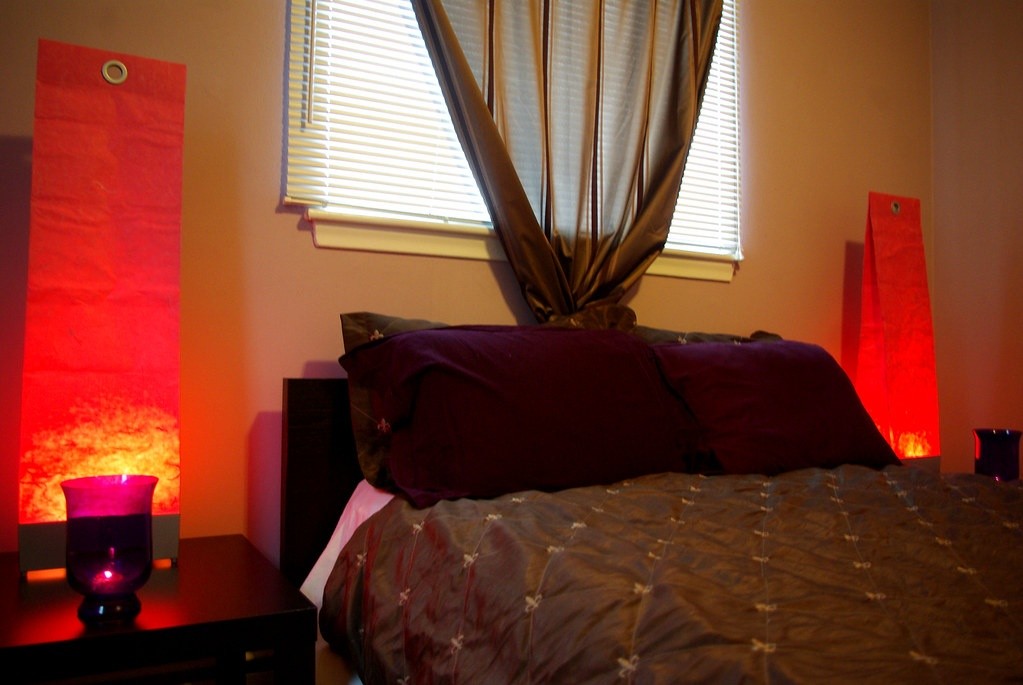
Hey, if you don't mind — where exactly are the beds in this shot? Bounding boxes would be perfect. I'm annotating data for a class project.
[281,376,1022,685]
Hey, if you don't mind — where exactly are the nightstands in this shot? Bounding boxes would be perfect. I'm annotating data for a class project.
[1,534,319,684]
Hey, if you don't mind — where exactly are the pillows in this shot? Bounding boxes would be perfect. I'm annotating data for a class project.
[649,339,902,474]
[342,310,685,509]
